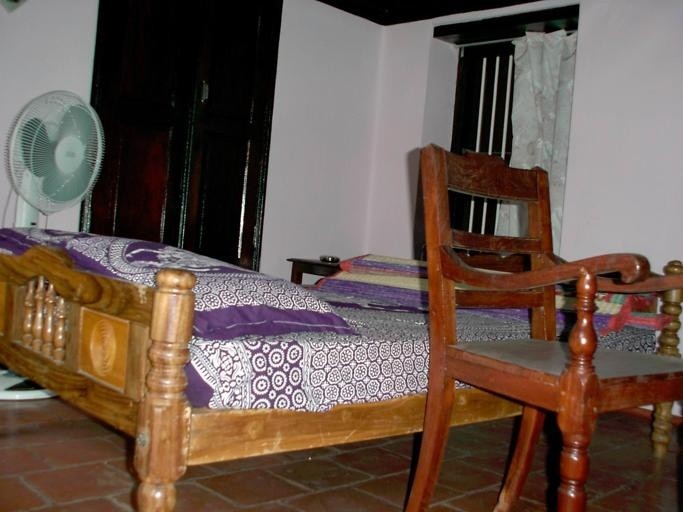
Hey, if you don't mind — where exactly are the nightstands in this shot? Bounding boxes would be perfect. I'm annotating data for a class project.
[286,258,342,286]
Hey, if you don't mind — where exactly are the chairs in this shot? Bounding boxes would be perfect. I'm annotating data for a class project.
[404,143,683,512]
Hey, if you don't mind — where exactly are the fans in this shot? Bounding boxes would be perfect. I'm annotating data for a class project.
[0,90,107,400]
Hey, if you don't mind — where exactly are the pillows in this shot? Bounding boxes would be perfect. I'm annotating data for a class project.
[0,225,682,511]
[29,228,360,341]
